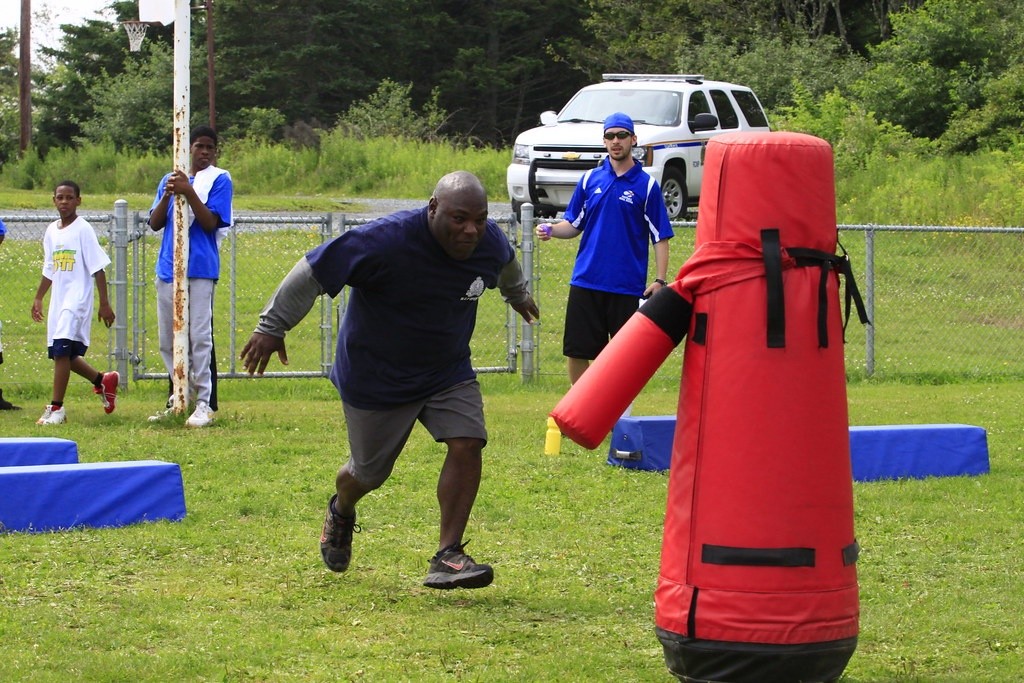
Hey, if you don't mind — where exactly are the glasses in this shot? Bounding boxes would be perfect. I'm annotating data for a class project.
[604,131,632,140]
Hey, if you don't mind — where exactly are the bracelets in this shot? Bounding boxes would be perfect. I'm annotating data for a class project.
[655,279,667,286]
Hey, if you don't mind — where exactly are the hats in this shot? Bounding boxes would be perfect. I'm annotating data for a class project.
[604,112,637,148]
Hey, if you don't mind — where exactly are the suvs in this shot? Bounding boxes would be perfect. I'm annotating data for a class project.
[504,72,772,223]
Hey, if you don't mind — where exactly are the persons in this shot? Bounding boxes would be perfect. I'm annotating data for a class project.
[535,112,675,388]
[31,181,120,426]
[238,170,540,590]
[147,124,235,431]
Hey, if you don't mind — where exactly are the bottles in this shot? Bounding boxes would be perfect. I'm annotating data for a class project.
[543,415,562,456]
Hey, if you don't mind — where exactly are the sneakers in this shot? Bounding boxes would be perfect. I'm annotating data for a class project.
[185,403,214,427]
[424,538,493,589]
[35,405,65,427]
[320,493,356,572]
[146,406,174,421]
[93,371,120,415]
